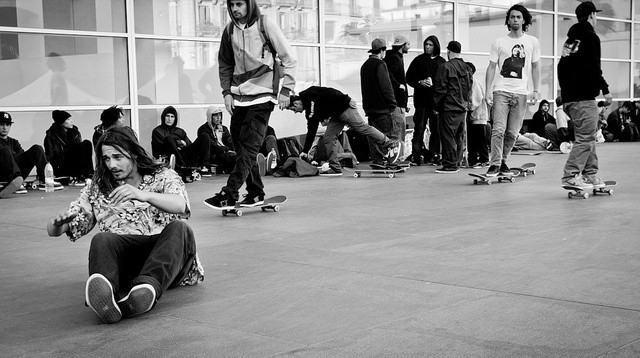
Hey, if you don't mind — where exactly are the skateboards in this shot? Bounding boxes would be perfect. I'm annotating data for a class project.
[562,181,619,197]
[344,167,405,178]
[469,170,520,183]
[222,195,287,216]
[510,163,536,177]
[559,142,574,154]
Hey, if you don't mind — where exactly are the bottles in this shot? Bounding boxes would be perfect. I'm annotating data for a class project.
[44,161,54,196]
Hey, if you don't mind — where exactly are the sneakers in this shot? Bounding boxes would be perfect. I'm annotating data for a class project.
[85,273,122,324]
[68,176,86,187]
[546,142,555,151]
[318,169,342,177]
[382,141,401,165]
[435,166,458,174]
[15,183,28,194]
[266,151,273,173]
[486,165,500,177]
[0,175,23,199]
[238,194,265,207]
[116,283,156,319]
[561,175,593,188]
[472,161,491,169]
[256,153,266,177]
[203,190,236,210]
[582,174,605,186]
[500,162,514,176]
[37,183,64,191]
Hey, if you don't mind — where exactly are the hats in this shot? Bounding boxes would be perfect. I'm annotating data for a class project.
[392,34,409,46]
[206,106,223,124]
[368,39,386,53]
[447,41,461,53]
[575,1,603,19]
[0,111,14,124]
[52,110,71,127]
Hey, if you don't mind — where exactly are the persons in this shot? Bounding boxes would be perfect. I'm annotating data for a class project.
[193,107,273,176]
[545,95,575,149]
[432,40,474,173]
[384,38,409,164]
[92,104,139,149]
[596,99,610,128]
[360,39,398,169]
[531,99,556,136]
[43,110,95,186]
[464,60,489,169]
[205,0,296,210]
[0,111,64,194]
[337,131,359,168]
[286,86,402,177]
[498,42,527,81]
[631,101,640,129]
[557,1,613,192]
[46,124,204,323]
[485,4,539,178]
[405,35,446,165]
[151,105,214,178]
[606,101,640,142]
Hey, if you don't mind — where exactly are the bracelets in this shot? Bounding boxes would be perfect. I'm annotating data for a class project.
[533,90,538,94]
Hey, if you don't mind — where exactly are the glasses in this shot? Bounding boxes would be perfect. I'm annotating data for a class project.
[626,107,630,111]
